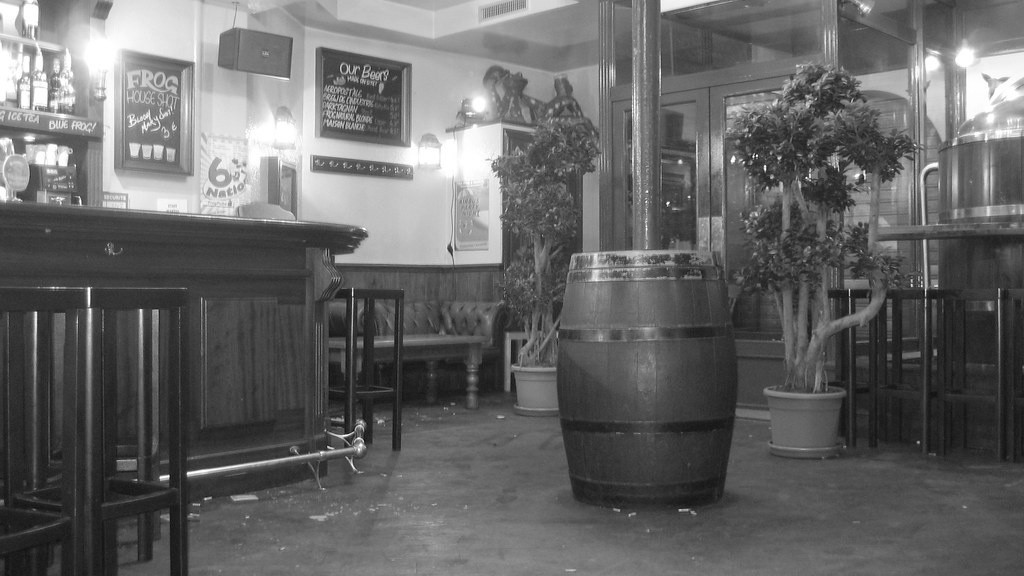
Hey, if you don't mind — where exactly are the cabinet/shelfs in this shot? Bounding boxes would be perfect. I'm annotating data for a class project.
[655,145,695,244]
[0,0,117,206]
[444,119,584,338]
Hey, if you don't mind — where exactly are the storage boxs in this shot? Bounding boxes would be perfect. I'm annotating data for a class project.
[47,192,72,205]
[18,164,79,194]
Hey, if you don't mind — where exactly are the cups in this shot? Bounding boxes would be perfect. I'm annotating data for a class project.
[25,143,73,165]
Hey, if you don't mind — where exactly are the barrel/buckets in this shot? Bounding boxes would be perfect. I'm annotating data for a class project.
[556,251,736,510]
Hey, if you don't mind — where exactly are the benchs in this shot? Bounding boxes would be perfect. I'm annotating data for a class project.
[328,295,510,397]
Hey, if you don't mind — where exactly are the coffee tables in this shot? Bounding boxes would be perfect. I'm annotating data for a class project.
[329,331,489,415]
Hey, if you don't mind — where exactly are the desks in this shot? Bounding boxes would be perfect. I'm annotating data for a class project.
[860,224,1024,445]
[0,197,372,504]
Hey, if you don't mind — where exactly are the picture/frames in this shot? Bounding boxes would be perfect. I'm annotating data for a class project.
[267,155,300,219]
[109,46,199,178]
[310,46,416,150]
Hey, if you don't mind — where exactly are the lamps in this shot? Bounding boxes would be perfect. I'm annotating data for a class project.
[458,94,494,120]
[265,106,304,152]
[414,131,444,174]
[978,71,1015,101]
[86,39,115,100]
[845,0,883,20]
[948,38,980,71]
[923,48,943,74]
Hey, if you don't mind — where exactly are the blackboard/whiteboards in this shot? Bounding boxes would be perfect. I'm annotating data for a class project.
[316,46,413,148]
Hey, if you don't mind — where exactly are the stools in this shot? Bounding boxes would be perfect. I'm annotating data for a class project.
[0,284,190,576]
[323,281,409,456]
[829,281,1024,463]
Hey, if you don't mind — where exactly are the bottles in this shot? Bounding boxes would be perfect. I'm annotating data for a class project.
[22,0,39,40]
[18,53,74,115]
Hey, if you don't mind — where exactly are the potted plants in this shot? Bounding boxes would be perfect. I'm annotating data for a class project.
[719,58,925,459]
[483,110,603,421]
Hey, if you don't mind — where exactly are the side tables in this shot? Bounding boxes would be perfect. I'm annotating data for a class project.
[499,329,570,398]
